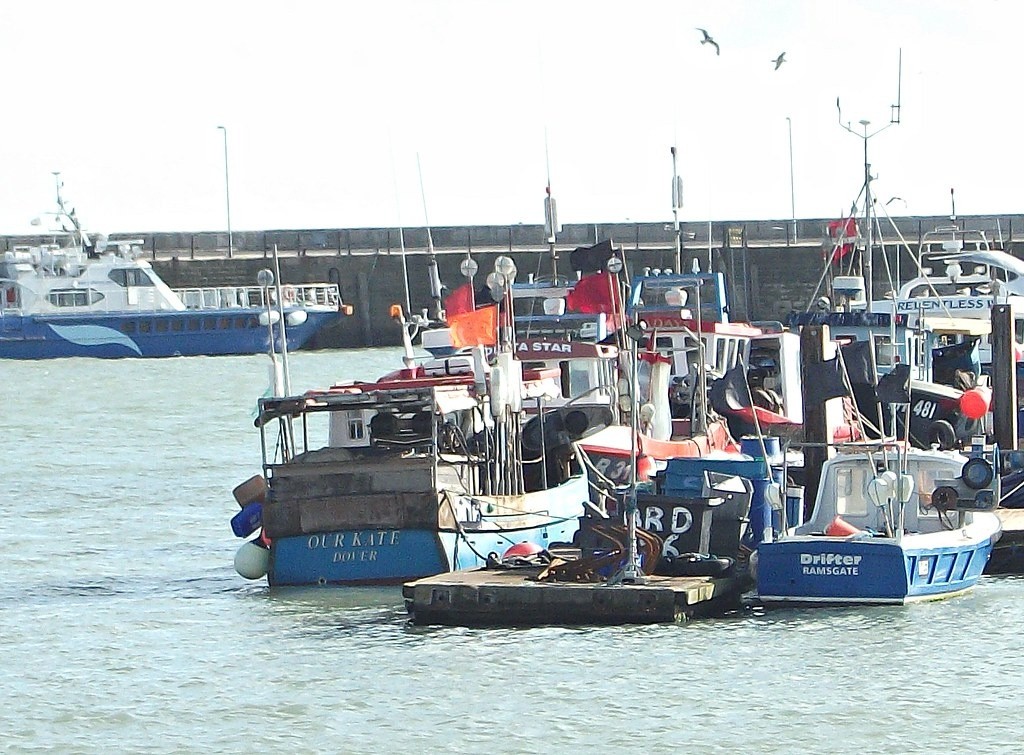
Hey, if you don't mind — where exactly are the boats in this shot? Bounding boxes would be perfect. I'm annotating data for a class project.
[231,46,1024,606]
[0,172,353,359]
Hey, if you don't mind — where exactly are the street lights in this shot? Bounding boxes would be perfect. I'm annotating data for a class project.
[218,127,232,259]
[785,117,795,244]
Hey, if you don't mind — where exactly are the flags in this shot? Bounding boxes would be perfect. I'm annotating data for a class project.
[709,364,751,412]
[564,270,619,314]
[802,355,851,404]
[444,283,473,319]
[474,284,510,326]
[836,341,877,385]
[447,306,496,347]
[872,364,911,403]
[569,238,612,271]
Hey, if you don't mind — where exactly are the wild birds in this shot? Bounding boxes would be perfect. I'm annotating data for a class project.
[771,52,787,70]
[695,27,720,55]
